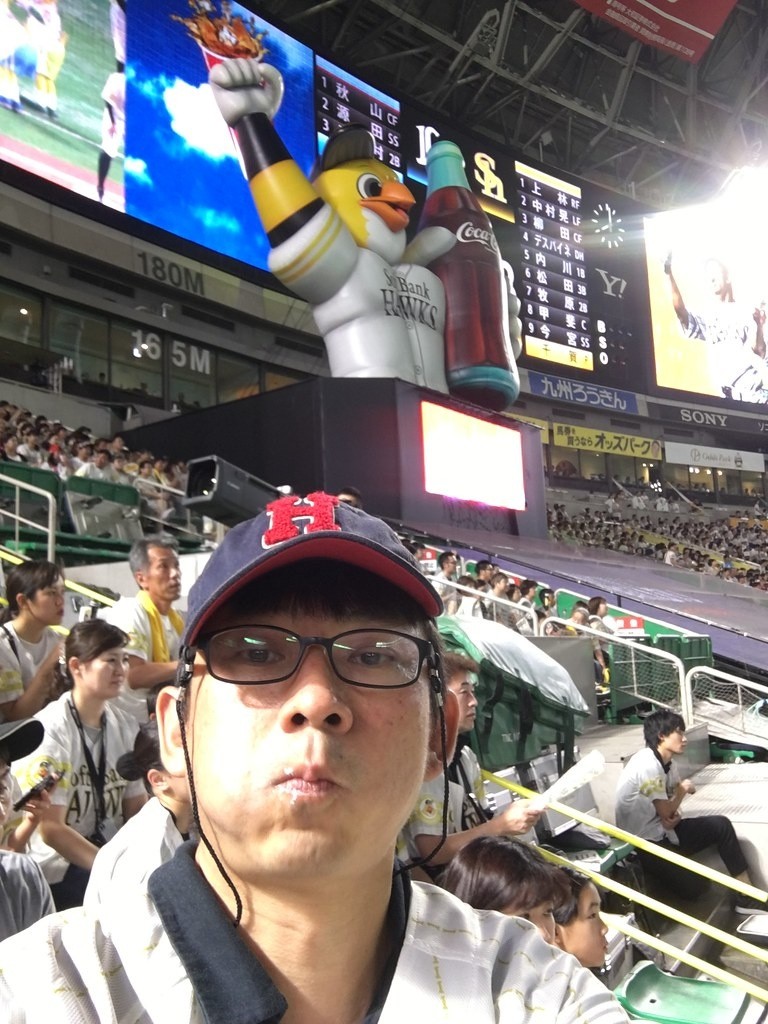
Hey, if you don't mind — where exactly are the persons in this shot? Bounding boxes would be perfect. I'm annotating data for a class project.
[0,371,768,1024]
[662,244,768,404]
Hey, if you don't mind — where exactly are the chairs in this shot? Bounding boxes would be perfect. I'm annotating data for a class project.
[0,459,768,1024]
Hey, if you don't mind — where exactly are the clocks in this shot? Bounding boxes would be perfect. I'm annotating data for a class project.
[590,201,630,250]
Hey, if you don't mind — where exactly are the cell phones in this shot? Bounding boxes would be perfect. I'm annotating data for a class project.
[13,776,54,811]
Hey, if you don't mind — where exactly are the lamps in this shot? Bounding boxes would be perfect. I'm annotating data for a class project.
[471,38,490,57]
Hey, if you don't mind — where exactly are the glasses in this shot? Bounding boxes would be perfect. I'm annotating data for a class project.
[193,623,438,689]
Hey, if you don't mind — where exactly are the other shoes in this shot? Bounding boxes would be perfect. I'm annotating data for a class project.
[735,892,768,915]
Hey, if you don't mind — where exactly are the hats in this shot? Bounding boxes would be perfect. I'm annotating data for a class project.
[183,492,444,649]
[0,719,44,762]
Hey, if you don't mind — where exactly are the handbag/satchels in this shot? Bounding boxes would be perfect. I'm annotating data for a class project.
[563,821,610,853]
